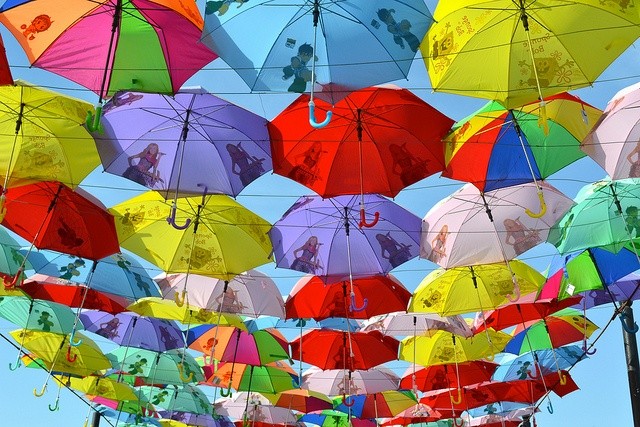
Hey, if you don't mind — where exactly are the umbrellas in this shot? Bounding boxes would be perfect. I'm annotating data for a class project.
[9,332,111,397]
[1,187,121,288]
[18,351,90,386]
[381,418,444,426]
[91,399,145,426]
[54,371,138,427]
[419,183,574,302]
[580,82,639,181]
[439,91,604,218]
[538,268,596,355]
[503,307,601,385]
[124,297,248,383]
[181,326,290,397]
[418,1,639,135]
[559,247,638,333]
[0,230,22,275]
[151,269,284,366]
[19,272,134,316]
[545,179,639,264]
[567,272,638,311]
[212,393,297,423]
[102,348,207,416]
[80,87,273,230]
[332,392,418,427]
[135,410,163,427]
[401,317,517,404]
[418,180,573,304]
[160,419,187,427]
[107,189,276,307]
[296,411,377,427]
[266,195,422,311]
[200,0,437,128]
[268,390,334,414]
[0,301,82,334]
[300,366,401,393]
[265,83,459,227]
[504,345,589,383]
[289,326,401,407]
[284,272,413,357]
[4,283,98,371]
[202,360,299,426]
[400,357,502,426]
[1,1,220,134]
[506,314,556,416]
[0,79,102,222]
[407,260,547,361]
[135,384,212,416]
[16,246,162,347]
[74,310,186,384]
[461,401,542,427]
[537,370,581,398]
